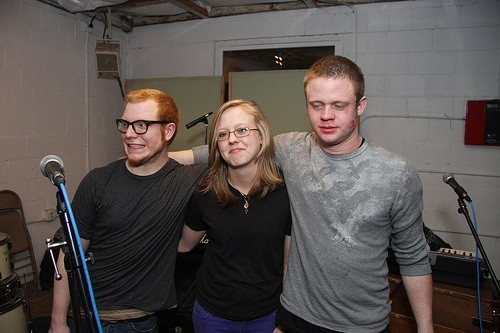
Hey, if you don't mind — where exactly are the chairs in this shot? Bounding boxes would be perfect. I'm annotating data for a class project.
[0,190,41,290]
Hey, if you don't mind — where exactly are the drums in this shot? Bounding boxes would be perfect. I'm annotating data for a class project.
[1,231,29,333]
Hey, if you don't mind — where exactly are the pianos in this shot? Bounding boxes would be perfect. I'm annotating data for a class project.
[383,244,492,291]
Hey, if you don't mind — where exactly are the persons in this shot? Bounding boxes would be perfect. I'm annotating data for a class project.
[48,89,210,333]
[117,56,433,333]
[178,98,293,332]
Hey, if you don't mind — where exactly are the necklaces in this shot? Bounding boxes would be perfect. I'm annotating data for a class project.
[229,178,249,215]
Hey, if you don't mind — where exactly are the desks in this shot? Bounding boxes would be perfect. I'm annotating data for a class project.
[388,274,500,333]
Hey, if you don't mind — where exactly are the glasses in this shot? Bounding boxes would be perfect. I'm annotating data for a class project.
[217,126,261,140]
[115,119,169,134]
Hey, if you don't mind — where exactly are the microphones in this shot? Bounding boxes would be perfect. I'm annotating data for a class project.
[39,154,66,188]
[186,111,213,129]
[443,173,472,203]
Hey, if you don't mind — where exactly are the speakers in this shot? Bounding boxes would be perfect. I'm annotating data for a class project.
[95,42,120,79]
